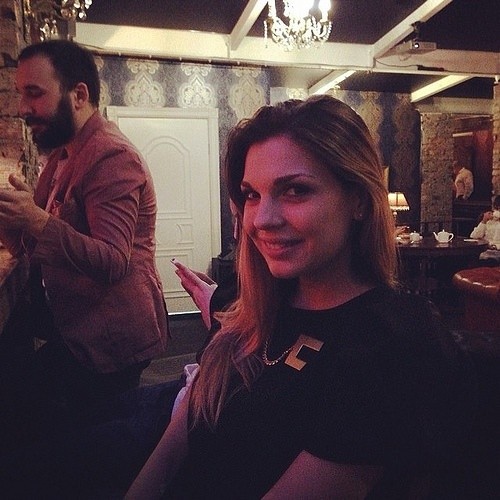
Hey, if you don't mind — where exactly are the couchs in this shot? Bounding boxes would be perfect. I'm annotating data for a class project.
[450,265,500,329]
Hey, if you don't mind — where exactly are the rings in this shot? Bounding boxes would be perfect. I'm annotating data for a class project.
[488,212,491,214]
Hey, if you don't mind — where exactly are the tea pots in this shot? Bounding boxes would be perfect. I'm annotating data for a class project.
[433,229,454,243]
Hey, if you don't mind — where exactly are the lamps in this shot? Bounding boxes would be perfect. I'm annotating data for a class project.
[387,191,411,229]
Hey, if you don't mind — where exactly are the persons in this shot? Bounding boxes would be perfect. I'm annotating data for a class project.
[470,194,500,267]
[450,162,475,199]
[0,40,171,500]
[122,94,435,500]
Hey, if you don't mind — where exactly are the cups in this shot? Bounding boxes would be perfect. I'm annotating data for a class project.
[410,233,419,241]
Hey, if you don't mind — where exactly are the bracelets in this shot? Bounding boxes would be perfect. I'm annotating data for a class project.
[493,245,495,249]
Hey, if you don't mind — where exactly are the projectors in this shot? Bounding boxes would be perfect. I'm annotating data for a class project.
[396,40,436,55]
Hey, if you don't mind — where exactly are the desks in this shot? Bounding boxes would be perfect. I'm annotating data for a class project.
[393,233,489,300]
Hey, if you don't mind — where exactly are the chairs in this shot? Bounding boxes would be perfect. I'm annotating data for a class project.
[57,339,500,500]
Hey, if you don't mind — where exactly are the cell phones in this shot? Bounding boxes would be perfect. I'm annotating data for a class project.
[170,257,187,269]
